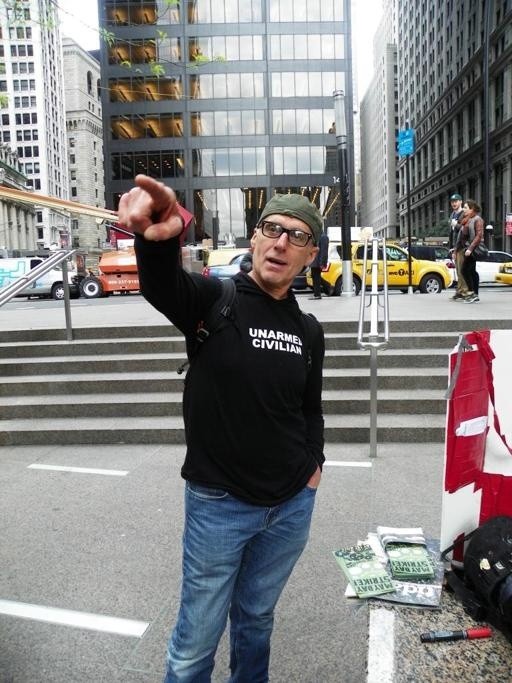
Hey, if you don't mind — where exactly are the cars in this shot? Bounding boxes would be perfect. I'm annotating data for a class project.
[305,242,452,295]
[199,255,309,291]
[495,261,512,285]
[401,245,512,288]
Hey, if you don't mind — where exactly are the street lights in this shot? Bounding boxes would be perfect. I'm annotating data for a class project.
[334,88,355,299]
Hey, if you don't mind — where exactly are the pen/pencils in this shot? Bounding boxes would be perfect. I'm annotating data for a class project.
[419,628,492,642]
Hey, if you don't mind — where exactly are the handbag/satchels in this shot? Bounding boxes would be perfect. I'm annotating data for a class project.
[466,240,489,260]
[440,516,512,643]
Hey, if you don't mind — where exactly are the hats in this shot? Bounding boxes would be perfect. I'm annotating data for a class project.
[257,193,322,244]
[450,194,461,200]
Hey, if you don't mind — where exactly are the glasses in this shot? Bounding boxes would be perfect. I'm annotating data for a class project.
[257,220,316,246]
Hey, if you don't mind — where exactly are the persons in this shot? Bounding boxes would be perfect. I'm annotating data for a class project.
[308,229,332,299]
[451,199,484,303]
[448,193,472,299]
[116,172,326,682]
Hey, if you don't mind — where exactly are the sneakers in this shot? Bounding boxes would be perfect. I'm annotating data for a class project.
[308,284,335,300]
[453,290,479,303]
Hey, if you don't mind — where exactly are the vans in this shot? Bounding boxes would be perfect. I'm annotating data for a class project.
[0,257,80,300]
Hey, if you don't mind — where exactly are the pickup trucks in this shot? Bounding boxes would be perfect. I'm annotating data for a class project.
[206,247,252,267]
